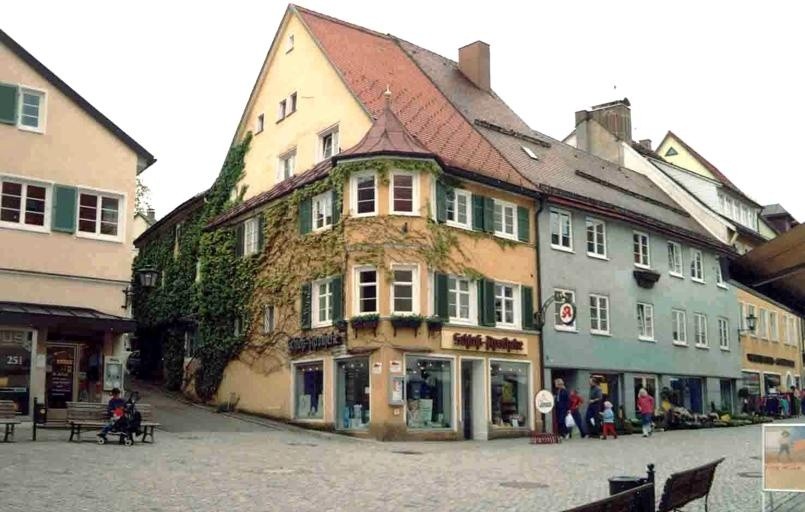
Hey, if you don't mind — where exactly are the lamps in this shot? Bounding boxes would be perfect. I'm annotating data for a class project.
[120,261,159,310]
[738,313,760,339]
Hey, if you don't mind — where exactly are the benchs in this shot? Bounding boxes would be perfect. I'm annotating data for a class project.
[64,399,162,445]
[657,454,725,512]
[0,398,22,442]
[563,462,657,512]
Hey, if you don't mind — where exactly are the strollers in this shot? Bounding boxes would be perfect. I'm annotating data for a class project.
[92,391,143,448]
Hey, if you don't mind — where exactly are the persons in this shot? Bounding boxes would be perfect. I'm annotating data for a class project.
[568,390,584,436]
[419,373,429,400]
[661,396,672,431]
[554,378,570,441]
[599,400,618,439]
[584,377,603,438]
[743,385,805,419]
[637,388,655,437]
[777,431,792,464]
[107,388,142,444]
